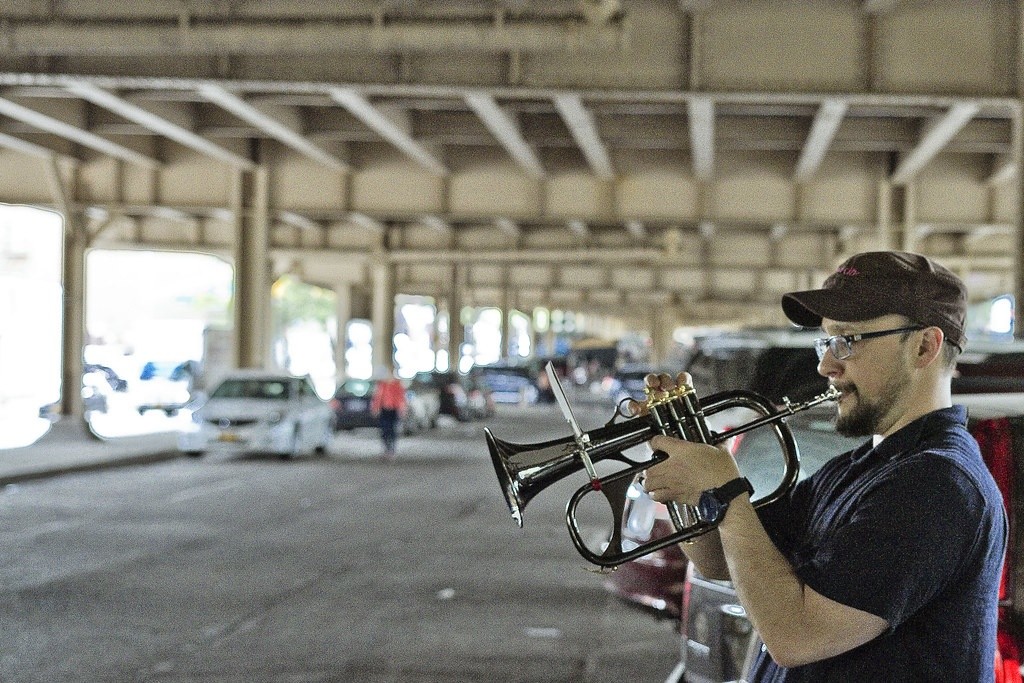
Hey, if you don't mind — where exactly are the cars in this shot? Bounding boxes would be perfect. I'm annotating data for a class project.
[177,373,331,462]
[597,328,1024,683]
[332,366,539,438]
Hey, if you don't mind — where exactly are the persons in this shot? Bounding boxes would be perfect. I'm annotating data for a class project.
[373,364,405,451]
[627,250,1010,683]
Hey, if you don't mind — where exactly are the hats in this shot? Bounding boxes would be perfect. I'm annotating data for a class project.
[780,251,971,354]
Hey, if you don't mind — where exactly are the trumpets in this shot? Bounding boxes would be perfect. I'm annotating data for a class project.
[482,361,847,573]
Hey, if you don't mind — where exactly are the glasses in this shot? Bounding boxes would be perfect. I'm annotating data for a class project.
[813,326,928,360]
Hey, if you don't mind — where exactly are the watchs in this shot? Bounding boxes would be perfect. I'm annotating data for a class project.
[698,477,753,523]
